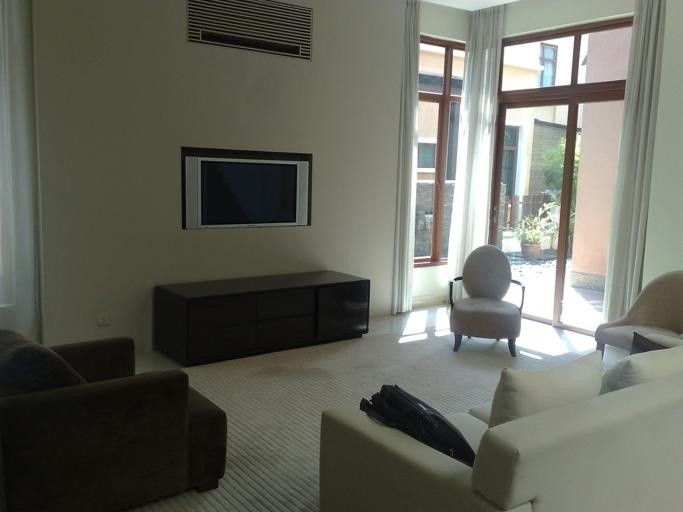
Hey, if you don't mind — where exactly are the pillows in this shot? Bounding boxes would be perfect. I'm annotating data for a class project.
[600,343,682,394]
[488,350,603,430]
[630,331,668,355]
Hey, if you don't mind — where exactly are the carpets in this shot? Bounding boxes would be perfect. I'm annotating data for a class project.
[125,331,494,512]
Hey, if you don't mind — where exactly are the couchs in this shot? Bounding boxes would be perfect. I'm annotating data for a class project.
[318,333,681,512]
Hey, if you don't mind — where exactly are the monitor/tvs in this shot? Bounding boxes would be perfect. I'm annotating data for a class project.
[185,156,309,229]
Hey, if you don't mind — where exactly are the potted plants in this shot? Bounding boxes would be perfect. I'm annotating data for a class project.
[506,215,550,260]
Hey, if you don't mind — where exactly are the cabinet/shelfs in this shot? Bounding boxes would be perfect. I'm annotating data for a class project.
[150,271,371,367]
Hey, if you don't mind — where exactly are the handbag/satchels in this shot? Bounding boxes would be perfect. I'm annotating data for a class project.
[358,383,476,467]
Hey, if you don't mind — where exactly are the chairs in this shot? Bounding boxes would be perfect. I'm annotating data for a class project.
[1,328,226,512]
[448,245,526,357]
[594,271,682,358]
